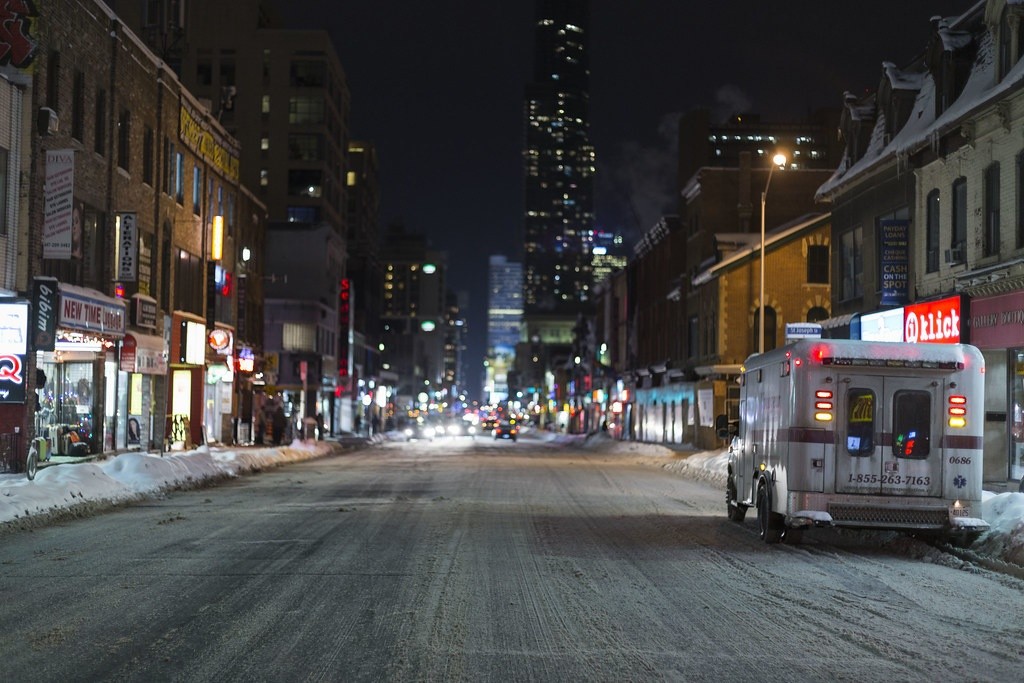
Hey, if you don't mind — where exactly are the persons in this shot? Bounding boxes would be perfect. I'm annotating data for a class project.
[316,413,324,441]
[272,407,286,445]
[128,418,141,441]
[354,414,404,435]
[256,405,267,445]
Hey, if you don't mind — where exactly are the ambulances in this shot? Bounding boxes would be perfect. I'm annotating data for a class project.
[715,337,992,546]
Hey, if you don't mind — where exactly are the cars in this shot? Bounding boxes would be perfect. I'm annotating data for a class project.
[405,407,523,443]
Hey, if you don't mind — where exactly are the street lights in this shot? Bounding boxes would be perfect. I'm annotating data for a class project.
[757,153,786,354]
